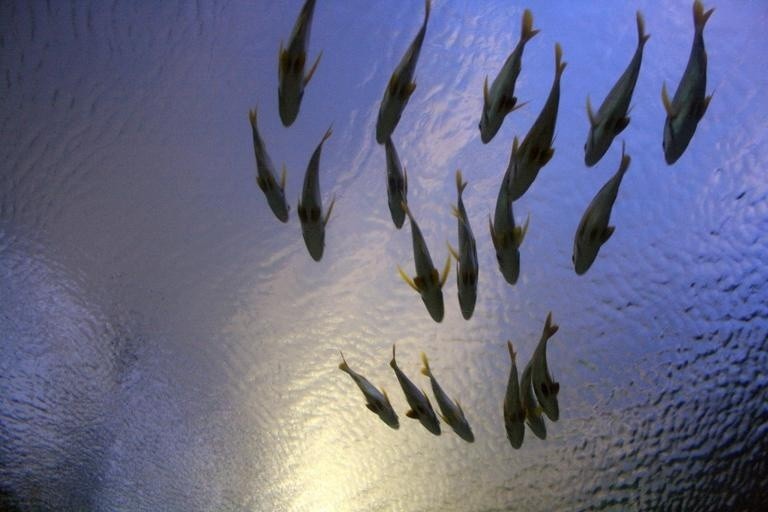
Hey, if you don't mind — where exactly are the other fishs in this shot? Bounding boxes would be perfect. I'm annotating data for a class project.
[478,9,543,144]
[531,310,560,422]
[571,140,632,276]
[296,118,337,262]
[247,99,292,224]
[501,41,568,200]
[583,10,652,167]
[520,354,547,441]
[421,351,474,444]
[277,0,324,127]
[388,344,443,434]
[660,0,717,166]
[385,137,407,228]
[503,338,526,450]
[488,135,529,285]
[376,0,432,144]
[395,161,452,323]
[337,350,401,431]
[446,168,480,321]
